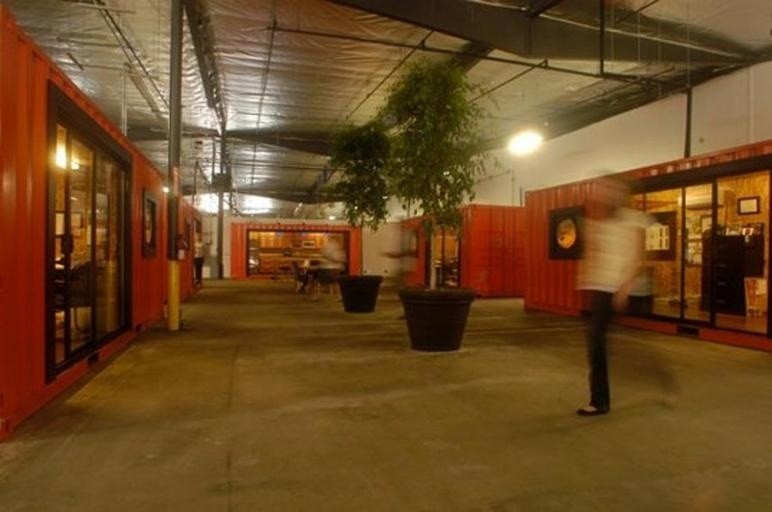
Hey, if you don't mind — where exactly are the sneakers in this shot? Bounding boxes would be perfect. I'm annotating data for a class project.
[577,406,607,416]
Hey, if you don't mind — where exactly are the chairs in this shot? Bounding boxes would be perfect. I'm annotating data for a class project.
[293,260,309,293]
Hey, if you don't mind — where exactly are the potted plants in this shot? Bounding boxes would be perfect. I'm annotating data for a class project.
[321,119,390,310]
[384,63,474,351]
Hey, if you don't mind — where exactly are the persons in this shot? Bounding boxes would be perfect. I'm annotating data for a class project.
[574,174,654,417]
[194,233,208,279]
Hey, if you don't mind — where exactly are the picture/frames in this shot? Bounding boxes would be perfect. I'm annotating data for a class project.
[547,206,586,260]
[142,186,157,256]
